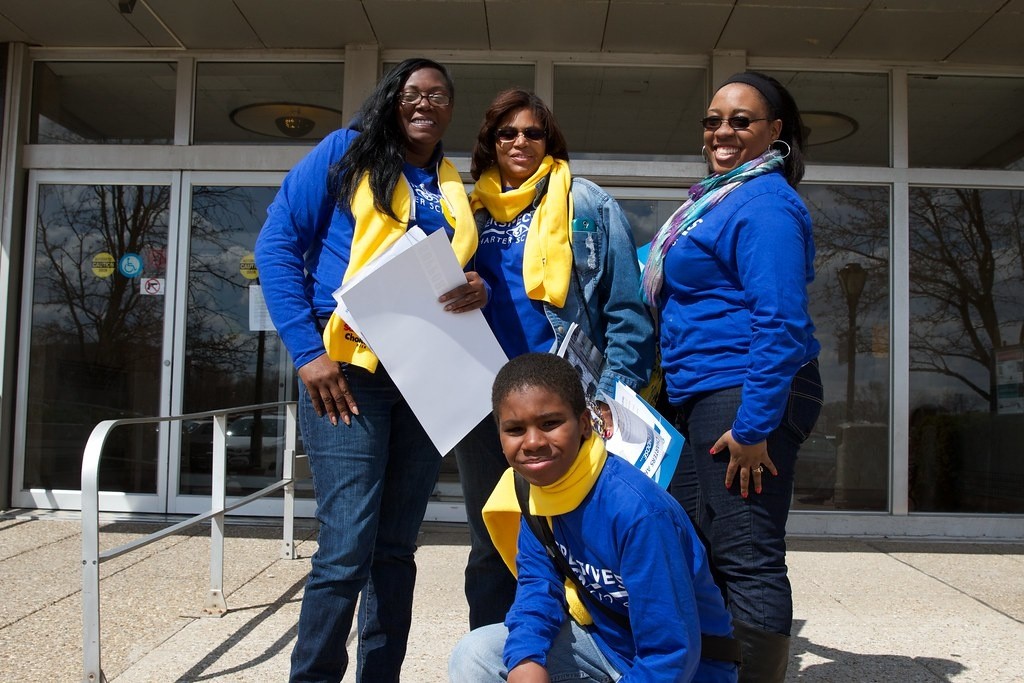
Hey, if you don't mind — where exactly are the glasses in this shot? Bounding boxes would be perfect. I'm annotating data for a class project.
[397,91,452,108]
[700,116,769,130]
[491,129,546,142]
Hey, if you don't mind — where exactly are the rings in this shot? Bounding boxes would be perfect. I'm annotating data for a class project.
[752,467,764,473]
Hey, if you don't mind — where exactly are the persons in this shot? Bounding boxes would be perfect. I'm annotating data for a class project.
[252,57,494,683]
[453,87,654,633]
[448,353,741,683]
[638,70,824,683]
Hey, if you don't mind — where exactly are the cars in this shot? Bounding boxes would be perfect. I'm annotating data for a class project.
[178,419,232,456]
[208,412,303,476]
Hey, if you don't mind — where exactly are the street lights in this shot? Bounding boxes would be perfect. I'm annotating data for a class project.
[835,262,871,506]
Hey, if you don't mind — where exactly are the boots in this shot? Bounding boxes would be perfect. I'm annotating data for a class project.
[732,619,790,683]
